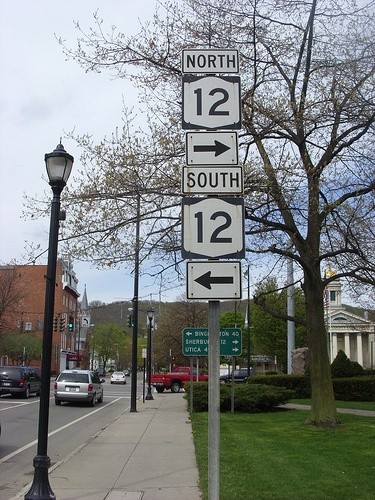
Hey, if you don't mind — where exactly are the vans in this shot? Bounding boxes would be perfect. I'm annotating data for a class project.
[96,368,106,377]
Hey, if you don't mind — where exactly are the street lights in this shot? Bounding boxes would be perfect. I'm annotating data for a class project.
[146,305,154,400]
[23,136,75,500]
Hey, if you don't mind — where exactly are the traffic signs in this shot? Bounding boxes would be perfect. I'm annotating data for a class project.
[182,327,243,357]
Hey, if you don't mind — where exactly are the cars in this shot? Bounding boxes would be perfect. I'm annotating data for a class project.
[122,370,130,376]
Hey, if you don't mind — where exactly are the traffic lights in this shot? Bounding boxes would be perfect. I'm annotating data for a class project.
[69,316,74,333]
[53,316,58,332]
[126,314,131,328]
[59,317,66,332]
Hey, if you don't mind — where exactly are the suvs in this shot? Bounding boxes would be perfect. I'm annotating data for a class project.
[220,368,256,384]
[54,369,106,406]
[110,372,127,385]
[0,366,40,399]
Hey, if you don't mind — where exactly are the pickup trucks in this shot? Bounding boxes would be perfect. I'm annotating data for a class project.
[149,367,208,393]
